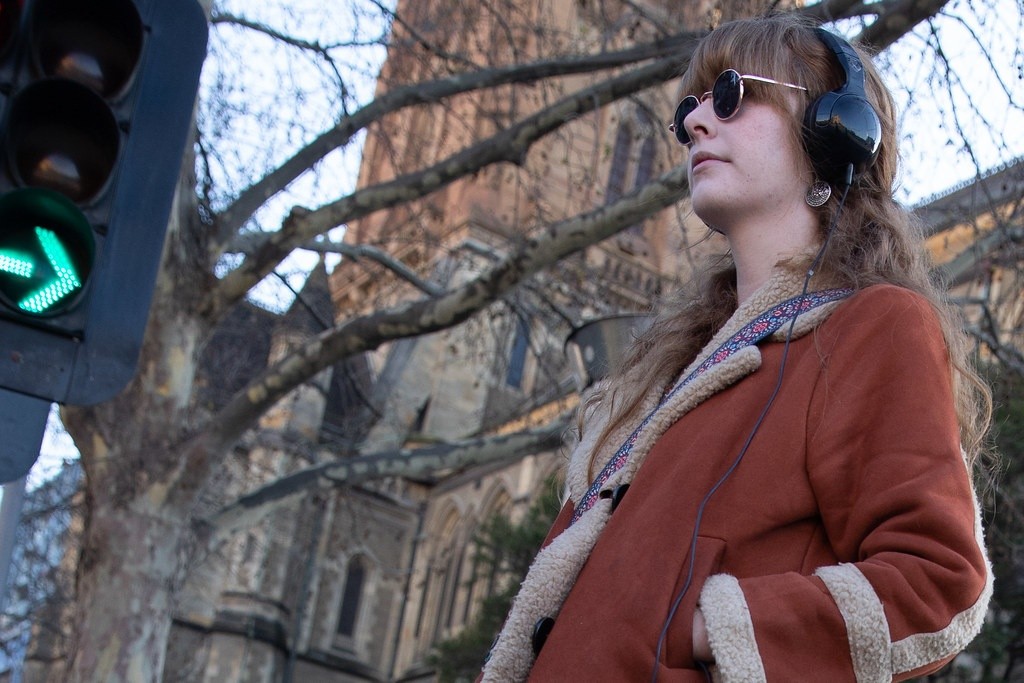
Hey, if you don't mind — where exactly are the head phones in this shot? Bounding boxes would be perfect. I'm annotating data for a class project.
[801,26,882,189]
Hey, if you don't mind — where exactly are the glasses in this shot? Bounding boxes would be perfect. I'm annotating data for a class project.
[668,69,811,146]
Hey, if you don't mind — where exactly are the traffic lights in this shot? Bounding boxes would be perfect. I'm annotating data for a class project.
[0,0,147,321]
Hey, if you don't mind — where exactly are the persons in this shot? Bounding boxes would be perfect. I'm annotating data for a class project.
[476,12,997,683]
